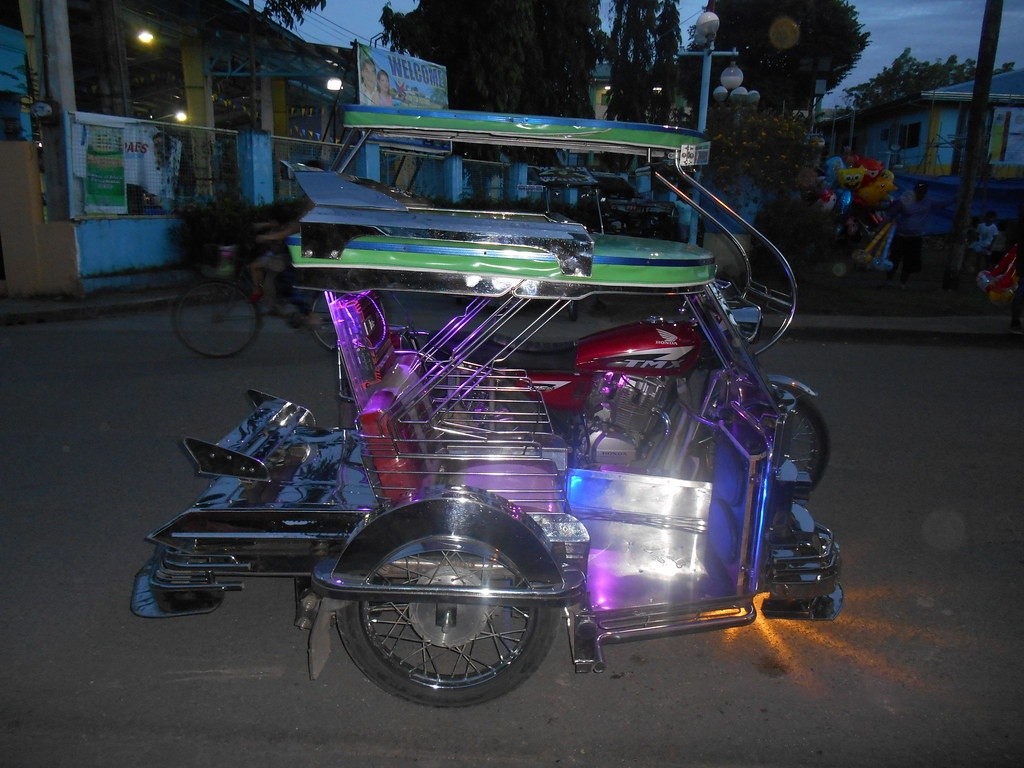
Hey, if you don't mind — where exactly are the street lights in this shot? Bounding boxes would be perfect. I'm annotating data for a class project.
[711,61,760,108]
[677,11,739,248]
[829,105,840,156]
[848,105,855,152]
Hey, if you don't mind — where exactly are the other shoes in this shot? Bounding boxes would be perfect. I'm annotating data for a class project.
[899,283,908,290]
[248,286,264,302]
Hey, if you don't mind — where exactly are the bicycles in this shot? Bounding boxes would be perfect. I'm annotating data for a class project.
[170,242,389,359]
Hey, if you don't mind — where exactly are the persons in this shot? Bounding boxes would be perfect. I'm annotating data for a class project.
[257,158,326,329]
[149,134,179,201]
[886,183,928,287]
[246,211,292,302]
[972,210,997,261]
[360,58,394,107]
[1010,200,1024,334]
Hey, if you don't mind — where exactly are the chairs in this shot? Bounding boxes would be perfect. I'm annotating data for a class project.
[358,354,564,516]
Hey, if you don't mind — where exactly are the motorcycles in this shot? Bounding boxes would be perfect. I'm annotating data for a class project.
[126,142,846,710]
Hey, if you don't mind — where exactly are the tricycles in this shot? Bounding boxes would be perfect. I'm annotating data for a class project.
[539,166,605,235]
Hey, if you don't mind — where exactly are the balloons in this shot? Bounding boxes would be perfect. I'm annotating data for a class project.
[817,149,897,243]
